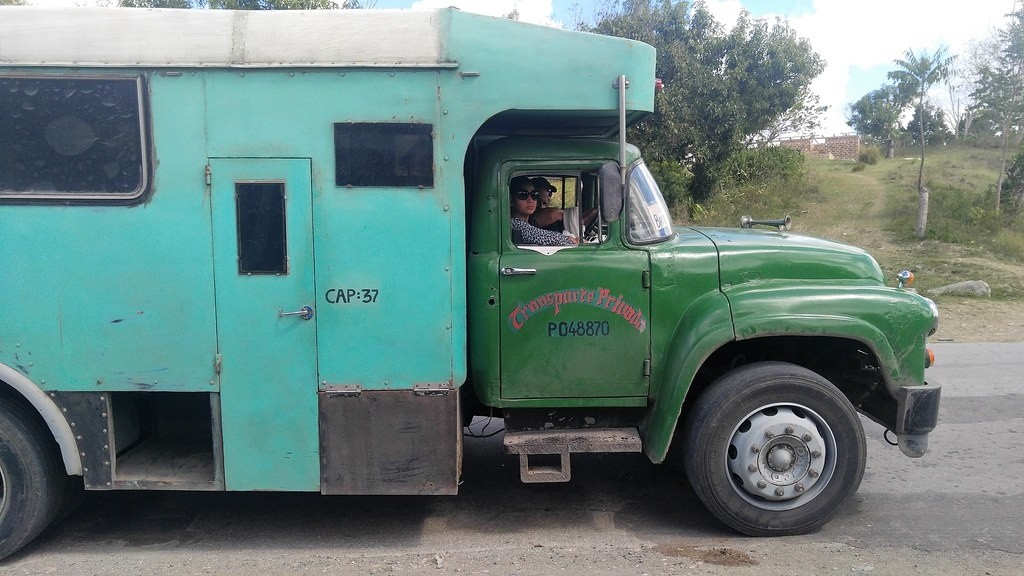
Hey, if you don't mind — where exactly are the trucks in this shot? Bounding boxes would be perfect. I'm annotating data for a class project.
[0,6,945,566]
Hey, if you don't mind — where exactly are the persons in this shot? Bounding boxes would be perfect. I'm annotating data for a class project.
[510,176,598,246]
[336,136,433,186]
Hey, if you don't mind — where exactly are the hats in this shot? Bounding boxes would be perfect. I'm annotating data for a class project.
[531,177,557,192]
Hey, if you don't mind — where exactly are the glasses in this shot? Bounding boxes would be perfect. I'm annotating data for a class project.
[513,190,540,200]
[541,186,552,193]
